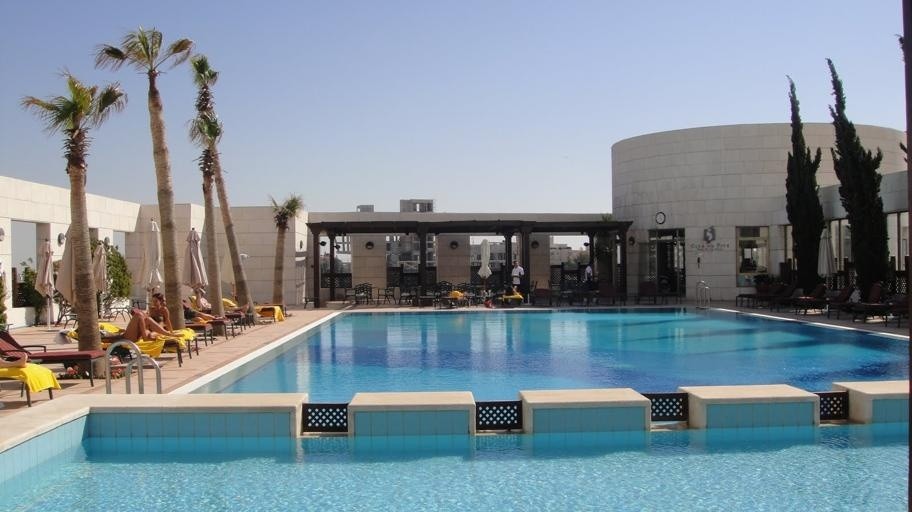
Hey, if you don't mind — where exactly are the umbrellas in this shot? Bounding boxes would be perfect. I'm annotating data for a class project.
[477,239,492,290]
[140,216,163,300]
[817,227,837,293]
[34,241,55,330]
[91,242,110,294]
[54,222,81,308]
[182,227,209,294]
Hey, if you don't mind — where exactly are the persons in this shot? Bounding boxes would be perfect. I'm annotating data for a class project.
[506,284,521,295]
[142,292,179,341]
[75,312,185,345]
[451,288,467,302]
[195,292,250,313]
[511,260,524,288]
[182,301,223,323]
[583,258,595,304]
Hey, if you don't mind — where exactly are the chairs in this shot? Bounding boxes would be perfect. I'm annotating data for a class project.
[343,279,681,308]
[736,281,910,329]
[1,295,284,407]
